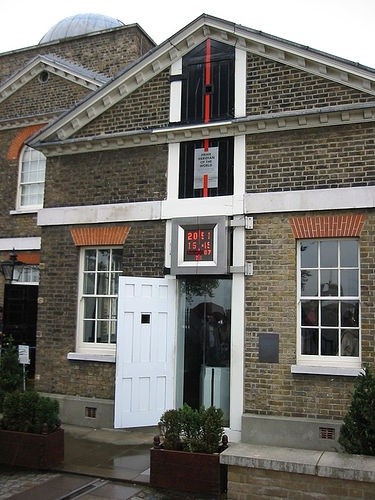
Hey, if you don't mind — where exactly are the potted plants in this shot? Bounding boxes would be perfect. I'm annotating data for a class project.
[0,390,67,471]
[148,402,228,496]
